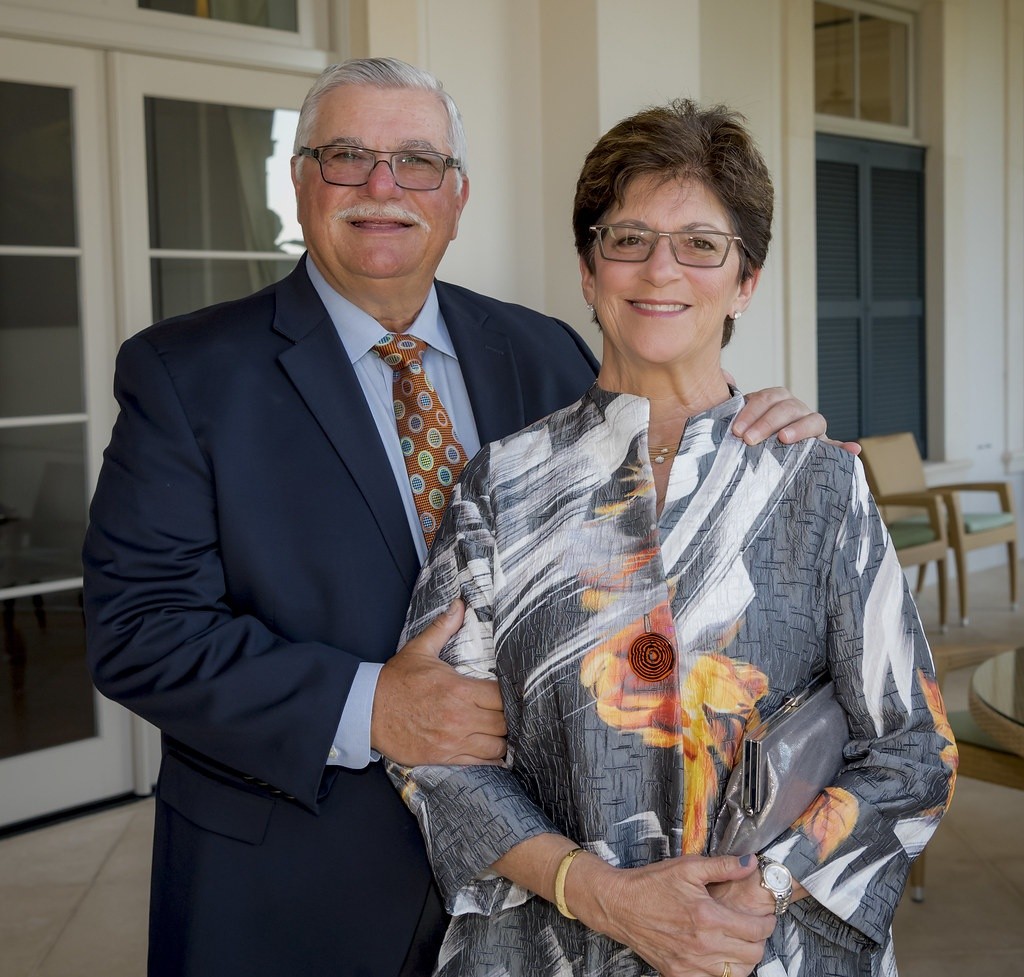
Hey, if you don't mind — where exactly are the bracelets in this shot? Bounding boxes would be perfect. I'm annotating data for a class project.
[555,847,586,920]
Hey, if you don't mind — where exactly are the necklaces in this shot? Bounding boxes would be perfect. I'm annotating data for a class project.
[649,443,678,464]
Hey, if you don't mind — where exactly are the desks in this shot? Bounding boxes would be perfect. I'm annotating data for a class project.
[970,649,1023,760]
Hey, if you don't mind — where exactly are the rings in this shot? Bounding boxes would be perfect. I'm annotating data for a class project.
[722,962,731,977]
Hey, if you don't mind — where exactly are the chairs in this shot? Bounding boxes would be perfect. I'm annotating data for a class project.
[911,644,1024,905]
[857,432,1018,634]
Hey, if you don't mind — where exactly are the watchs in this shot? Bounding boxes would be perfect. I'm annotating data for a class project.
[757,855,793,916]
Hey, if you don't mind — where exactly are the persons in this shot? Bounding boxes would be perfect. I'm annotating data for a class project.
[82,56,863,976]
[377,100,957,977]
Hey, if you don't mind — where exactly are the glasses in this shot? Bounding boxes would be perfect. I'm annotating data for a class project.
[300,144,459,191]
[588,224,762,268]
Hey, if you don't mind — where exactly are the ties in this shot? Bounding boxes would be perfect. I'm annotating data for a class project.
[370,332,470,553]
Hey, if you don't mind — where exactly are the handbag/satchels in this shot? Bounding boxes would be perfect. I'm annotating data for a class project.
[704,669,854,858]
